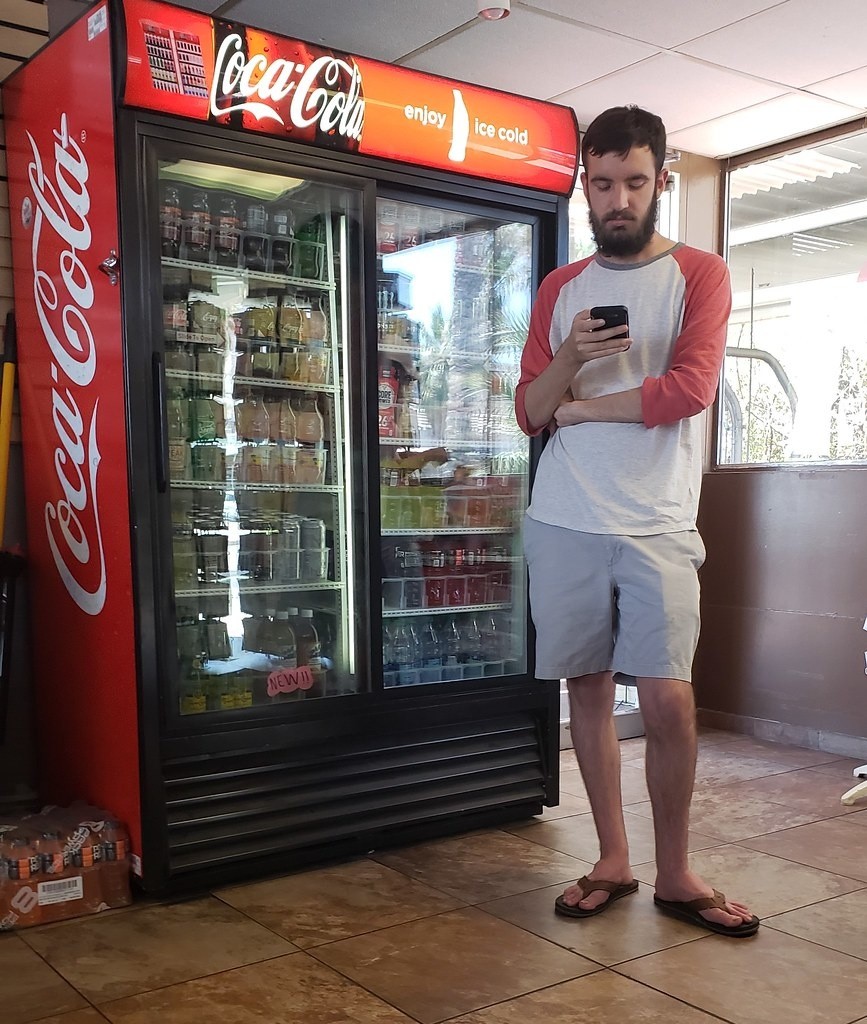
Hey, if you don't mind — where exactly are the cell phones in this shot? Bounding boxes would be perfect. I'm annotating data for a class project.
[590,306,631,352]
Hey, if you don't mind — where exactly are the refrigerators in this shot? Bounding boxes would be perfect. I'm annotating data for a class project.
[2,69,580,889]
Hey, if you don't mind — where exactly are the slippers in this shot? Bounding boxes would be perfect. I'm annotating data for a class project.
[653,888,759,937]
[555,874,639,919]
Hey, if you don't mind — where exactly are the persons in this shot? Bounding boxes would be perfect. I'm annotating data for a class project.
[380,334,426,670]
[514,107,760,938]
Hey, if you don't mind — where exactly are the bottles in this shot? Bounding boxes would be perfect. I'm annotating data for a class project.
[161,188,527,709]
[0,797,132,931]
